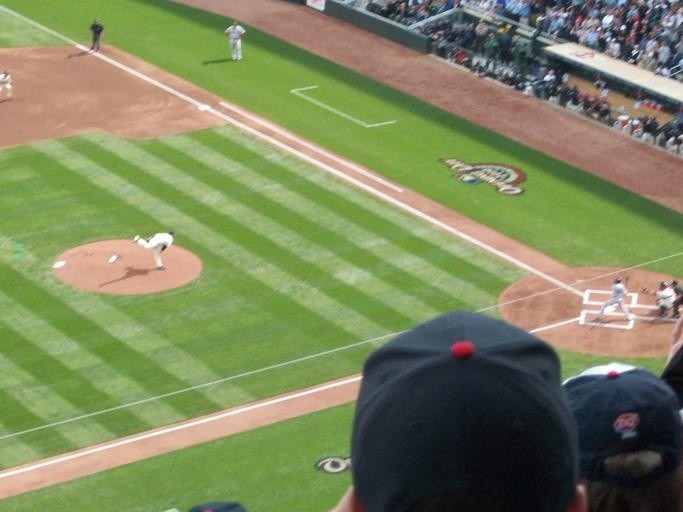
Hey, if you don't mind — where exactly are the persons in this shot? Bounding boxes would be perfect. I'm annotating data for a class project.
[0,70,13,101]
[133,230,174,270]
[90,18,102,51]
[365,0,682,159]
[594,280,683,320]
[332,312,682,511]
[224,22,245,61]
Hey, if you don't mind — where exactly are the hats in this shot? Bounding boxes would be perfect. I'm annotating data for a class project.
[350,310,578,510]
[561,363,683,485]
